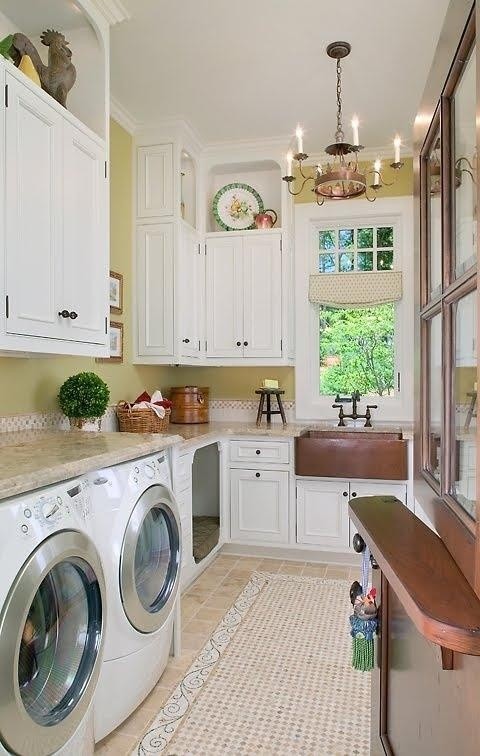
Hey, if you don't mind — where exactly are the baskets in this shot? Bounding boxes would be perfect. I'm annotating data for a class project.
[116,398,171,433]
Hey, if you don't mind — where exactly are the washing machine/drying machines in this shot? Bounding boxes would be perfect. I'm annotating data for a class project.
[85,449,184,745]
[0,474,109,755]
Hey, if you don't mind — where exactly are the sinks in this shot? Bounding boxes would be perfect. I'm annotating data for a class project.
[295,429,408,480]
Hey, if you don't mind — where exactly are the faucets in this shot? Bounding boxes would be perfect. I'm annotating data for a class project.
[351,391,361,414]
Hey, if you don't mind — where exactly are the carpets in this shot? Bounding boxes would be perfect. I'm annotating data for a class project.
[128,569,377,756]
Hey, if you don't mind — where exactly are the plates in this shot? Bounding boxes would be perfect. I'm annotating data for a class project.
[211,183,264,231]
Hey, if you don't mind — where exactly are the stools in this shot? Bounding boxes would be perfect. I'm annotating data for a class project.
[255,389,288,426]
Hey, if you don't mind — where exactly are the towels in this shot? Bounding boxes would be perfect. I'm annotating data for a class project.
[124,389,172,420]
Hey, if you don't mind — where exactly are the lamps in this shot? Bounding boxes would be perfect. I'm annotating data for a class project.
[285,42,405,208]
[426,123,476,199]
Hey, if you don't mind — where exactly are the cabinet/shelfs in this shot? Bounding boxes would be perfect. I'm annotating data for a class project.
[230,429,296,551]
[0,56,110,359]
[203,229,295,368]
[130,116,203,227]
[296,474,410,557]
[460,435,478,500]
[135,216,203,367]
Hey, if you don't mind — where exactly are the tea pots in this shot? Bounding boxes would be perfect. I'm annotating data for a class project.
[253,208,278,229]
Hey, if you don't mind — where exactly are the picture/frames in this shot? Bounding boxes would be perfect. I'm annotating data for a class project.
[96,322,124,363]
[109,270,124,317]
